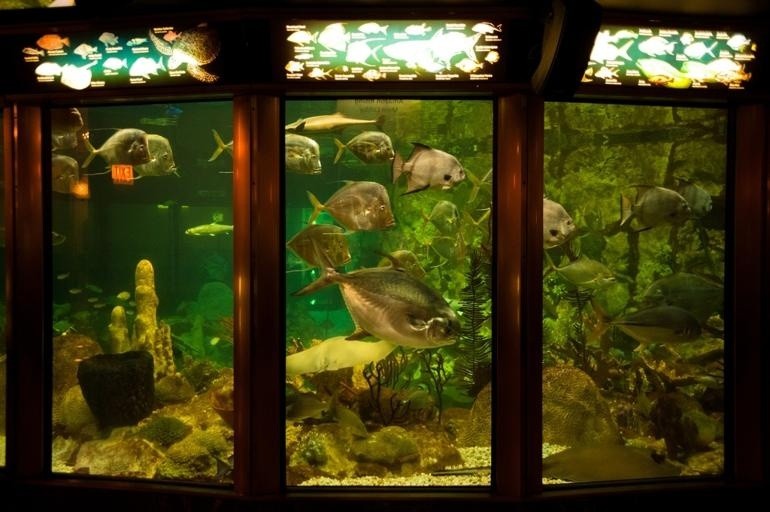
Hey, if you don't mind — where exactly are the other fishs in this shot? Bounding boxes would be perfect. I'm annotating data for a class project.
[278,110,469,351]
